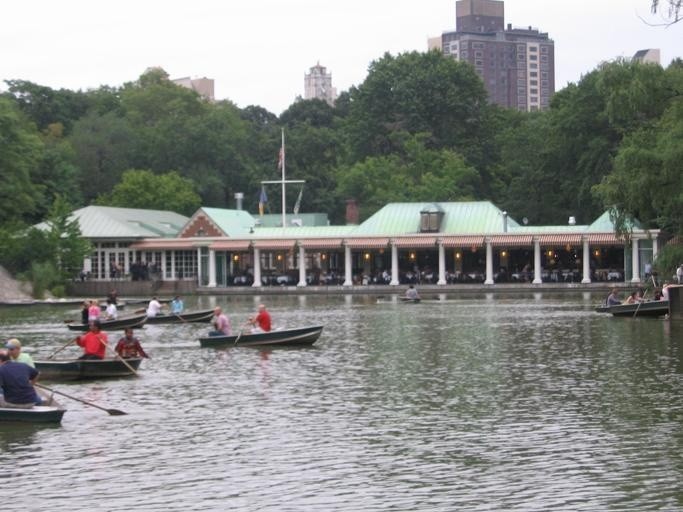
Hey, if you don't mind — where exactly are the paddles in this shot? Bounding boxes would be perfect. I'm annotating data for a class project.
[33,382,128,415]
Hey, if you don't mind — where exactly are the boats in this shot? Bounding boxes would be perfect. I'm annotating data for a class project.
[67,313,147,331]
[136,296,178,305]
[198,323,324,349]
[594,297,669,318]
[101,301,127,310]
[29,355,145,379]
[0,385,68,423]
[143,307,215,325]
[398,296,421,304]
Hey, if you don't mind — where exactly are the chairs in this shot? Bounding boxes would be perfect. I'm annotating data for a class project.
[223,263,623,287]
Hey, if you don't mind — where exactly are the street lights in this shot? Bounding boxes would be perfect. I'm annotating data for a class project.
[234,192,245,211]
[567,215,577,225]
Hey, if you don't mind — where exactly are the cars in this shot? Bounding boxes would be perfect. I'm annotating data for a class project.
[233,267,298,286]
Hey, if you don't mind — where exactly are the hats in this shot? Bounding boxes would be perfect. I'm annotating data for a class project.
[5,338,21,349]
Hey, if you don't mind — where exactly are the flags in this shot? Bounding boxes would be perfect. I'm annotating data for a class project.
[293,192,303,217]
[258,190,267,219]
[276,144,283,169]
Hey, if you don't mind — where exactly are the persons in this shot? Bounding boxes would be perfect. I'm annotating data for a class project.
[247,304,271,333]
[82,302,89,323]
[208,307,232,336]
[169,296,184,315]
[115,327,151,359]
[105,299,118,320]
[147,296,167,317]
[76,321,108,360]
[0,349,42,409]
[87,301,101,322]
[0,338,35,395]
